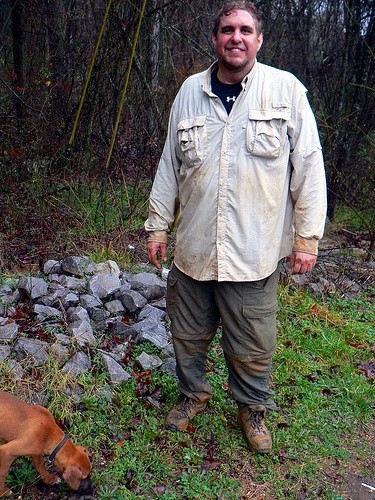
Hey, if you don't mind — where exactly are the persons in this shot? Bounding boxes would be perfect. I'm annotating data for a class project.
[144,0,327,454]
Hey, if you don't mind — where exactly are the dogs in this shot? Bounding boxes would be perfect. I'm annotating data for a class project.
[0,387,93,500]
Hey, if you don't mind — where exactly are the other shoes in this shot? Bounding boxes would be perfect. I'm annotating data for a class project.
[239,407,272,453]
[163,393,206,432]
